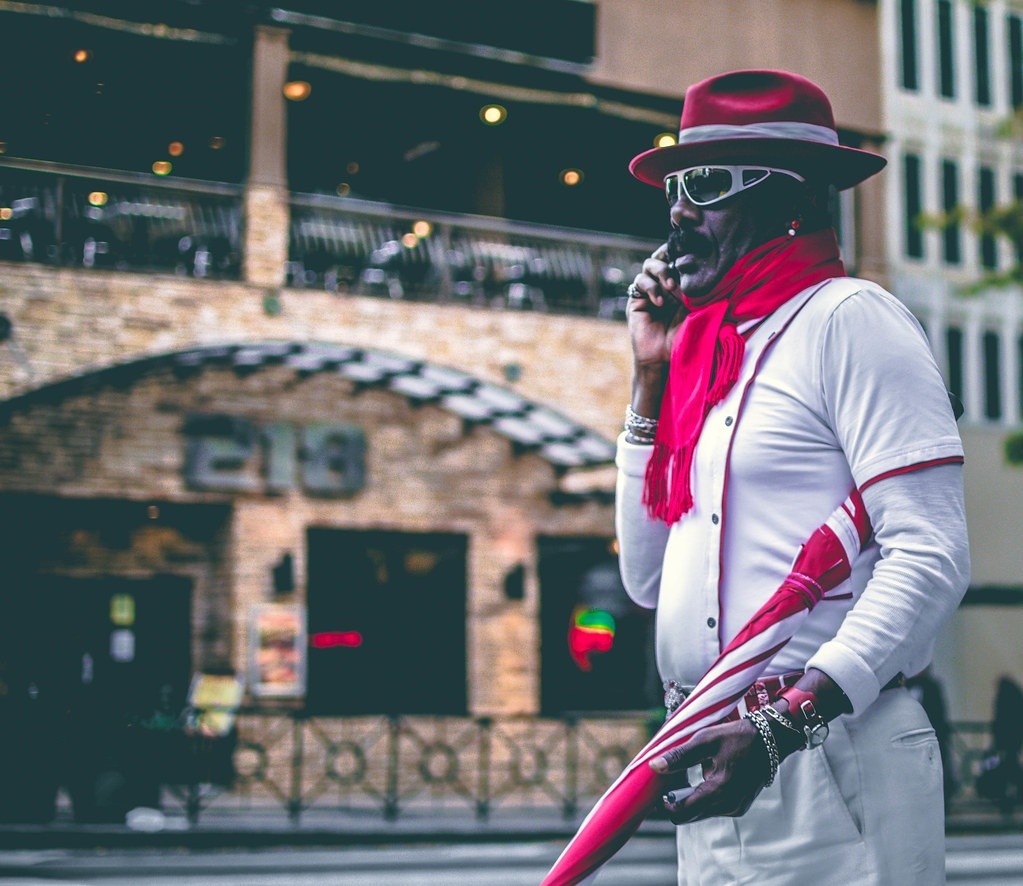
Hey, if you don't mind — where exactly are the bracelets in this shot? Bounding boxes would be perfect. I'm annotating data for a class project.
[743,711,779,787]
[763,705,800,733]
[625,405,658,442]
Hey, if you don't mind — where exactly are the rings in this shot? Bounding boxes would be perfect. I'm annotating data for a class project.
[628,284,644,298]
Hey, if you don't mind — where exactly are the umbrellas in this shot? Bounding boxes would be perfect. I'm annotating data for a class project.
[538,392,964,886]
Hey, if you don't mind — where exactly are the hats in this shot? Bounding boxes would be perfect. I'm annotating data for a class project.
[629,69,887,193]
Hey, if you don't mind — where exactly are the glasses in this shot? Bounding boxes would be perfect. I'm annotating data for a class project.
[664,164,805,207]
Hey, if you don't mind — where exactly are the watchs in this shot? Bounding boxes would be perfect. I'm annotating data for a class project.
[775,686,829,750]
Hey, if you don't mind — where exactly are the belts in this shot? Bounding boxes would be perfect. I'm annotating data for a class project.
[662,665,904,724]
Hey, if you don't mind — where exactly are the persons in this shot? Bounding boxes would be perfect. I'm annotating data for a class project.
[615,69,971,886]
